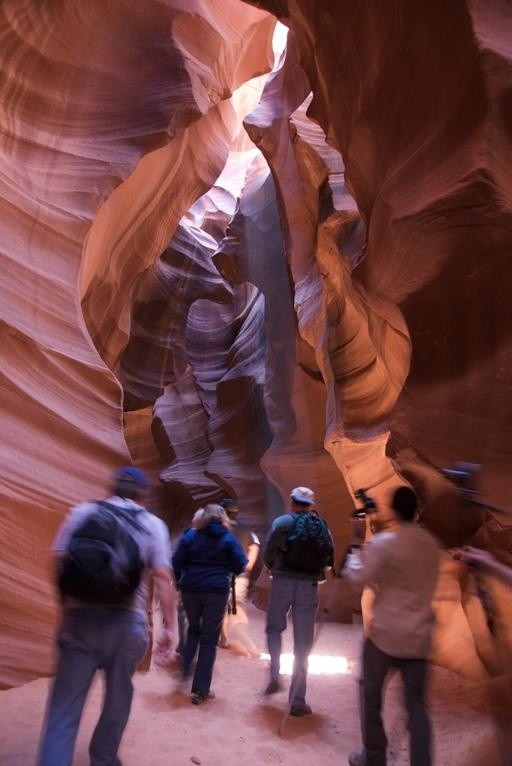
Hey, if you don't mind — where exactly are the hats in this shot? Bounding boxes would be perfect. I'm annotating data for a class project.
[290,486,317,506]
[117,467,148,485]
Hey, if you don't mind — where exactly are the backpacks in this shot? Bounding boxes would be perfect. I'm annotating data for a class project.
[280,511,333,573]
[59,497,149,607]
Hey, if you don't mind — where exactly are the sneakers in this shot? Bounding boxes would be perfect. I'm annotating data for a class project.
[267,679,280,693]
[349,751,386,766]
[290,704,312,716]
[189,689,216,705]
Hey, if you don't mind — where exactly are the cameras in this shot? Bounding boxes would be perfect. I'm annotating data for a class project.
[349,489,378,519]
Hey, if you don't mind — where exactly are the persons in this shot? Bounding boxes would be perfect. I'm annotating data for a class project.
[452,545,512,588]
